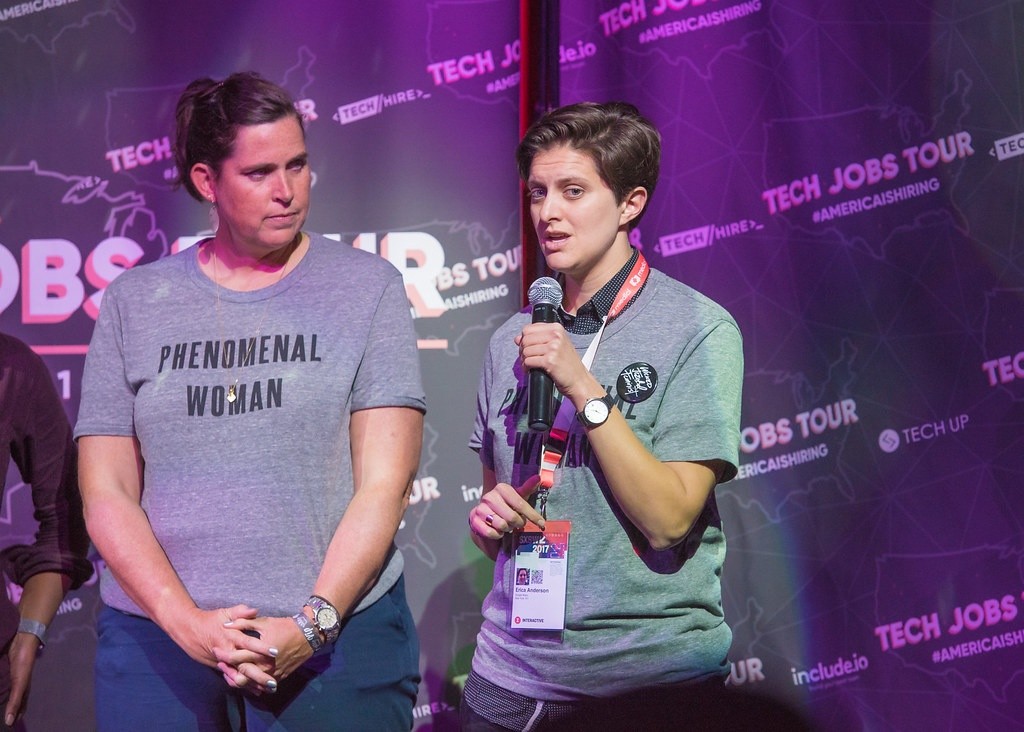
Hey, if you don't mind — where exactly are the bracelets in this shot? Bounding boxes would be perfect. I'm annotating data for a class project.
[309,595,343,624]
[18,618,49,652]
[292,612,324,653]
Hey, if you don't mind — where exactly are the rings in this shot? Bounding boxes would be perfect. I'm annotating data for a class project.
[485,513,496,526]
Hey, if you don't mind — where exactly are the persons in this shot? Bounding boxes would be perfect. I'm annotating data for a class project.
[462,98,745,732]
[70,72,429,732]
[0,332,96,732]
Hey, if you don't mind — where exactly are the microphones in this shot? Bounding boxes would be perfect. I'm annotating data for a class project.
[526,277,562,431]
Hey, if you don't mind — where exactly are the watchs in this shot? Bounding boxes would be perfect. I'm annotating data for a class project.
[307,595,341,647]
[575,393,614,427]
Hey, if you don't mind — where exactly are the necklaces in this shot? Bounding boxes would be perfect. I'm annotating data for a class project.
[213,231,294,404]
